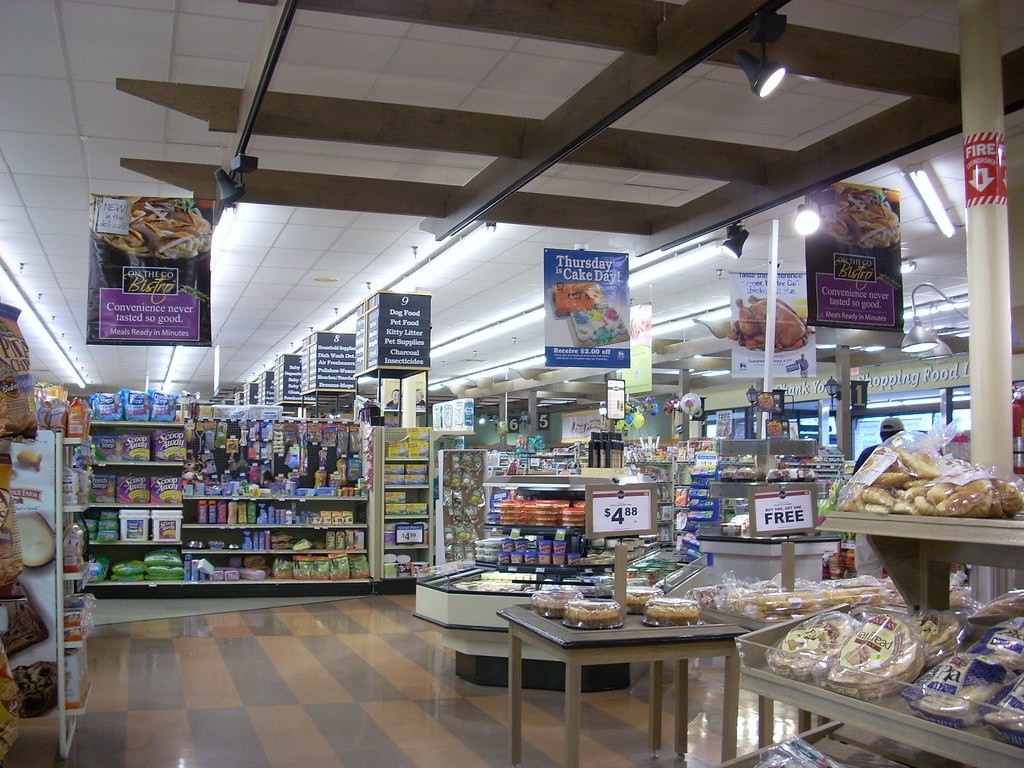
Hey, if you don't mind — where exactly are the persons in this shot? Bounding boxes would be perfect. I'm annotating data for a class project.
[416,388,425,406]
[384,389,399,409]
[852,417,905,477]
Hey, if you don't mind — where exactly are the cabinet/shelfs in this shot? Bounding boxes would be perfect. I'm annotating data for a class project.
[718,512,1024,768]
[0,427,93,760]
[413,438,858,692]
[84,421,433,598]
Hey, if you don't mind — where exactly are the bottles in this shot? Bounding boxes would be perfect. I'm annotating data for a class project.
[338,487,360,497]
[242,531,270,550]
[198,500,297,526]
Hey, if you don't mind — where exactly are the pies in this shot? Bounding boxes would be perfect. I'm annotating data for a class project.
[766,588,1024,749]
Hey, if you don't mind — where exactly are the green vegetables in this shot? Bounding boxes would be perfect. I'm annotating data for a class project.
[163,198,202,216]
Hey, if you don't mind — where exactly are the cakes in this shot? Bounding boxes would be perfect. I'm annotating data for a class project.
[551,281,608,316]
[531,588,702,628]
[568,306,630,347]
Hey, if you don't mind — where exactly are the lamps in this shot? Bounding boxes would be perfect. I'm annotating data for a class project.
[900,283,968,362]
[735,12,788,98]
[521,410,531,430]
[493,413,502,432]
[900,261,917,274]
[825,376,842,406]
[796,187,840,235]
[746,385,757,411]
[721,220,749,260]
[907,164,957,238]
[214,155,259,209]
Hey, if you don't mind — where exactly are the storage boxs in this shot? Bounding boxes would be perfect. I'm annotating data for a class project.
[386,432,430,577]
[91,428,187,504]
[64,599,90,709]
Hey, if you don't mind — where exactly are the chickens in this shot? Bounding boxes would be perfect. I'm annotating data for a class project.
[730,294,808,350]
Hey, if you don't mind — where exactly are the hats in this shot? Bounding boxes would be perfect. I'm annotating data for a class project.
[880,417,904,432]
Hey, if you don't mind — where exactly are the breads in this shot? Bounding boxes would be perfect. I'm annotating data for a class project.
[840,447,1024,520]
[686,575,965,622]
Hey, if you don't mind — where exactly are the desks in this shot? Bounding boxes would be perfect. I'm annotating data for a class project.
[496,603,832,768]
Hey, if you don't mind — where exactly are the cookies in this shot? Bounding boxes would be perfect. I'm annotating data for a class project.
[0,582,49,656]
[0,660,62,719]
